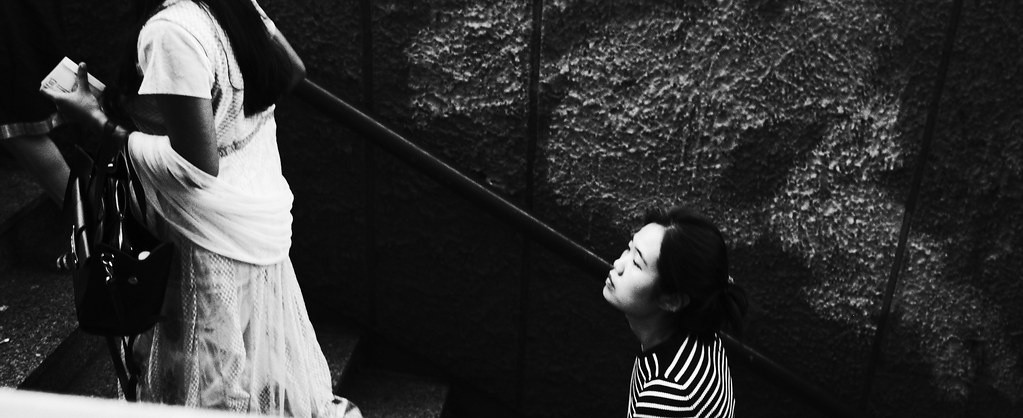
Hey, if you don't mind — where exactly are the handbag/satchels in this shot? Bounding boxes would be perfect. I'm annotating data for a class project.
[64,120,169,337]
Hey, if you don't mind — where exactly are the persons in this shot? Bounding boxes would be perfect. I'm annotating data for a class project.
[40,0,333,418]
[0,0,71,208]
[602,211,746,417]
